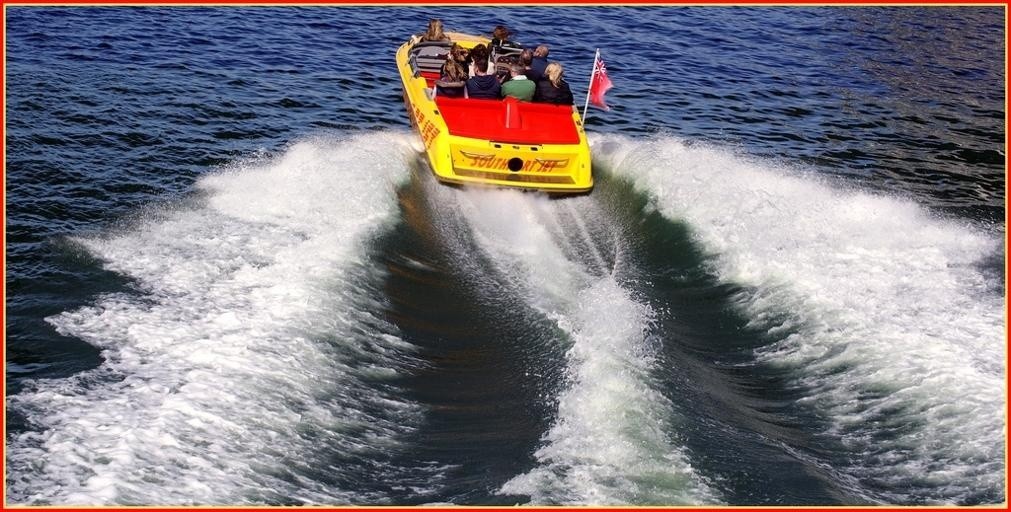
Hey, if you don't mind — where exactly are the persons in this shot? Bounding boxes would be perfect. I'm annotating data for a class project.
[466,56,502,99]
[468,44,497,80]
[439,60,469,84]
[501,61,537,103]
[501,48,539,84]
[533,62,575,106]
[488,25,517,54]
[413,19,452,44]
[527,44,550,81]
[440,43,470,75]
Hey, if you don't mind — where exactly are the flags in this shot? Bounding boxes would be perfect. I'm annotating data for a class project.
[589,53,616,112]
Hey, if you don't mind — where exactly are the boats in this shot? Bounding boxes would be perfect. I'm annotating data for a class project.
[397,31,596,197]
